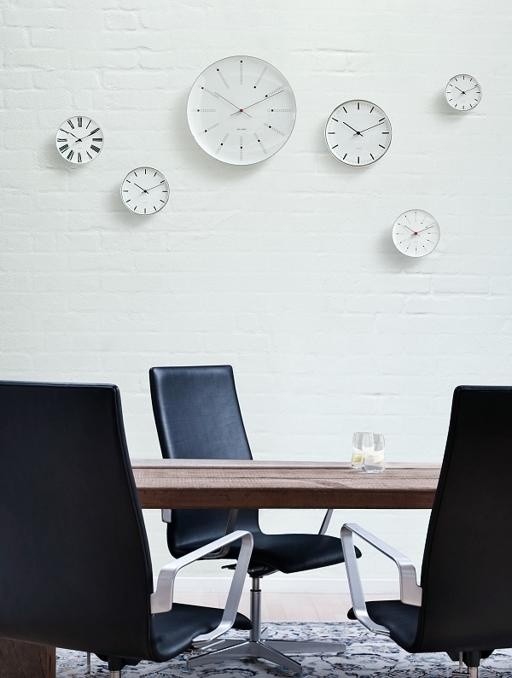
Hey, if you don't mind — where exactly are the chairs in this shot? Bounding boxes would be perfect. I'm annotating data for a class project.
[149,366,362,676]
[339,386,511,678]
[1,380,255,678]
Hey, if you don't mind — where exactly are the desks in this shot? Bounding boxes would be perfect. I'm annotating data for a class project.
[124,454,443,514]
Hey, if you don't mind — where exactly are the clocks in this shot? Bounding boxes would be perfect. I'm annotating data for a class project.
[184,55,297,167]
[54,115,105,165]
[445,74,482,112]
[324,99,394,167]
[391,208,440,258]
[120,167,169,216]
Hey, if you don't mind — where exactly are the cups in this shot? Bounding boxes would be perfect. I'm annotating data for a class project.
[350,432,388,473]
